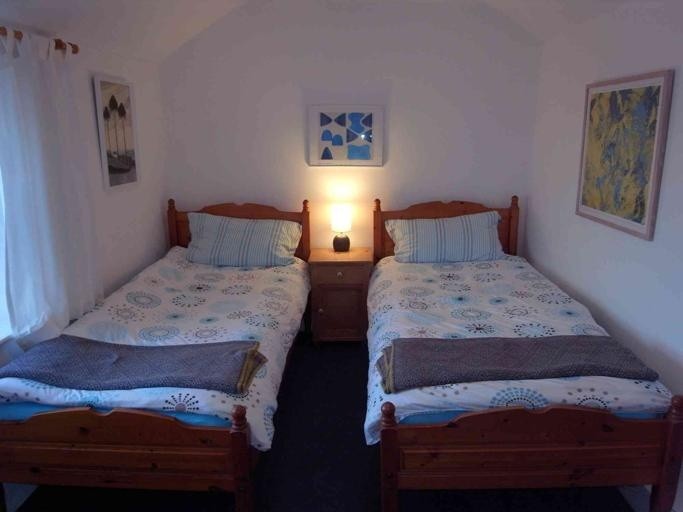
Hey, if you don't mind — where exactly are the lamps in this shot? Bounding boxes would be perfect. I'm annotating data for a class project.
[328,203,352,252]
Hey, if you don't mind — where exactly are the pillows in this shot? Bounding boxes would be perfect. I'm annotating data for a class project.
[185,212,303,266]
[385,209,506,263]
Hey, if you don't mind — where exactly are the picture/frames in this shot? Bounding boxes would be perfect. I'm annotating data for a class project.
[306,103,383,167]
[575,69,675,242]
[91,72,142,193]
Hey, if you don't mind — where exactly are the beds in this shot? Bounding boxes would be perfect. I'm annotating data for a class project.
[363,195,683,511]
[0,197,311,512]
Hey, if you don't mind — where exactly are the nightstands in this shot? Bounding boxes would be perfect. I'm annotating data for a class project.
[305,246,374,348]
